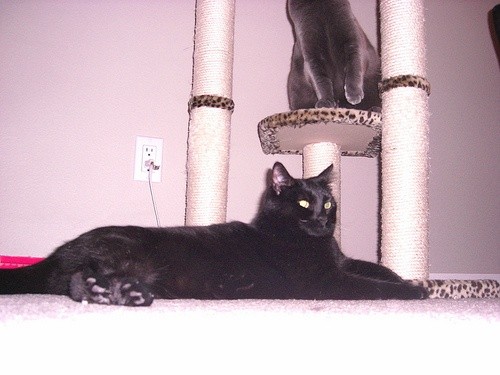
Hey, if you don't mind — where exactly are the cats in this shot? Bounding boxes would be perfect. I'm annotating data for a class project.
[285,0,383,113]
[1,162,428,306]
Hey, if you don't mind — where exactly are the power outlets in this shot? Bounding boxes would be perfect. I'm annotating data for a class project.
[133,136,163,182]
